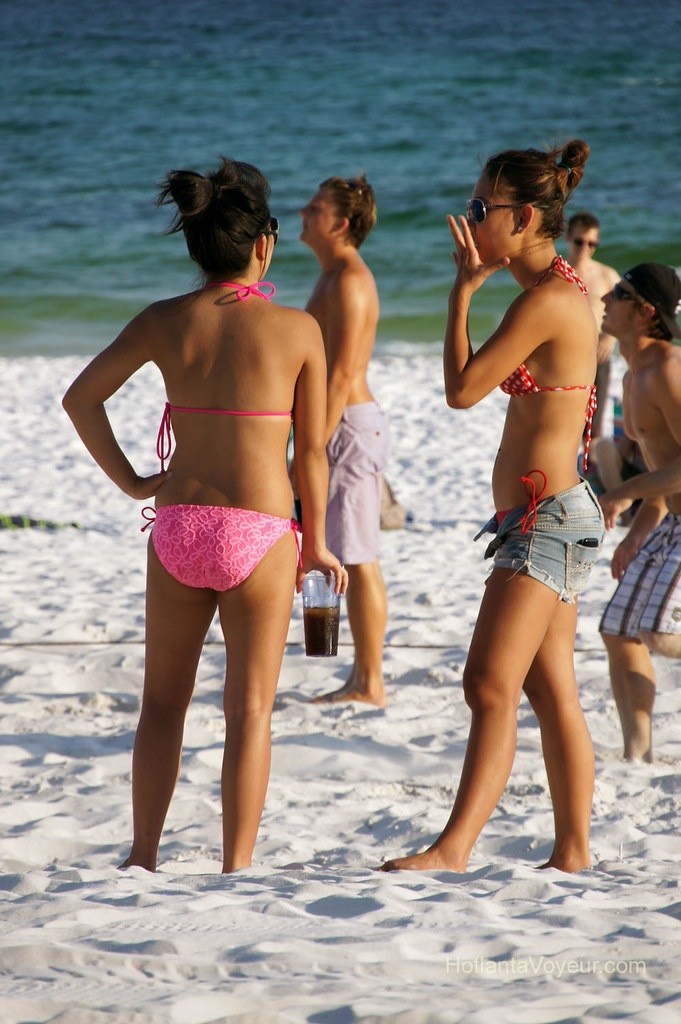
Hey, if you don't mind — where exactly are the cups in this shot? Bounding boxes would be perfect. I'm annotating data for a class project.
[301,575,341,657]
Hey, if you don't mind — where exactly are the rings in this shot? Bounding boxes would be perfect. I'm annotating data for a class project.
[298,175,393,710]
[340,565,344,571]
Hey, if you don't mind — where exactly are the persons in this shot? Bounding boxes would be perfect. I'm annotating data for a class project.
[375,142,606,874]
[59,156,349,880]
[556,214,628,462]
[599,261,681,763]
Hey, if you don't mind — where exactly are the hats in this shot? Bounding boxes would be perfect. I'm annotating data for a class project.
[622,262,681,341]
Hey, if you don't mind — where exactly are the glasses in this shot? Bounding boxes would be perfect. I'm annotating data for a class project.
[465,197,550,224]
[257,216,279,245]
[612,283,639,304]
[570,235,601,249]
